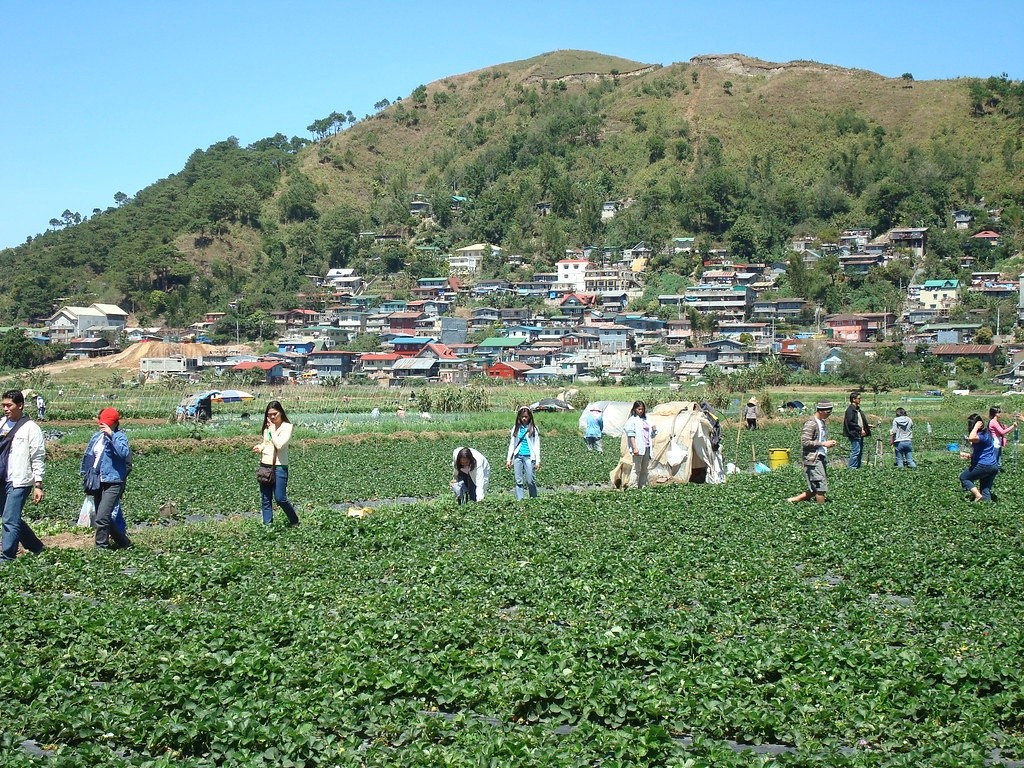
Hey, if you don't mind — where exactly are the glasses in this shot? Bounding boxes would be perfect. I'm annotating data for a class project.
[997,411,1001,413]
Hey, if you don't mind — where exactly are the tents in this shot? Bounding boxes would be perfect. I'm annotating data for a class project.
[530,398,576,412]
[178,391,221,422]
[577,401,726,485]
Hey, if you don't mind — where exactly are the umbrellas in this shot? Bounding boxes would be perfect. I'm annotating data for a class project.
[210,390,255,419]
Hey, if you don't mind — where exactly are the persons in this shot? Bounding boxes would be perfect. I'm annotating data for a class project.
[788,400,835,504]
[452,447,490,504]
[624,401,658,490]
[891,408,917,468]
[959,414,998,503]
[32,394,45,421]
[744,397,758,430]
[396,407,405,417]
[270,390,274,398]
[253,401,299,525]
[411,391,415,397]
[81,407,132,548]
[371,408,381,418]
[988,405,1017,464]
[507,406,541,500]
[251,390,255,396]
[842,393,871,467]
[0,389,45,559]
[586,407,603,452]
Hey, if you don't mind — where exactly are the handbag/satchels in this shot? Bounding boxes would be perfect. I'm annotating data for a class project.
[84,467,101,494]
[77,495,96,528]
[257,466,275,485]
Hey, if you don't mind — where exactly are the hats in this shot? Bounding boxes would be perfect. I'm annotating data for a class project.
[747,399,756,406]
[817,400,833,409]
[98,408,119,427]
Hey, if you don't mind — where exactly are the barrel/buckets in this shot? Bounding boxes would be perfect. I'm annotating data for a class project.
[947,443,959,452]
[769,448,791,471]
